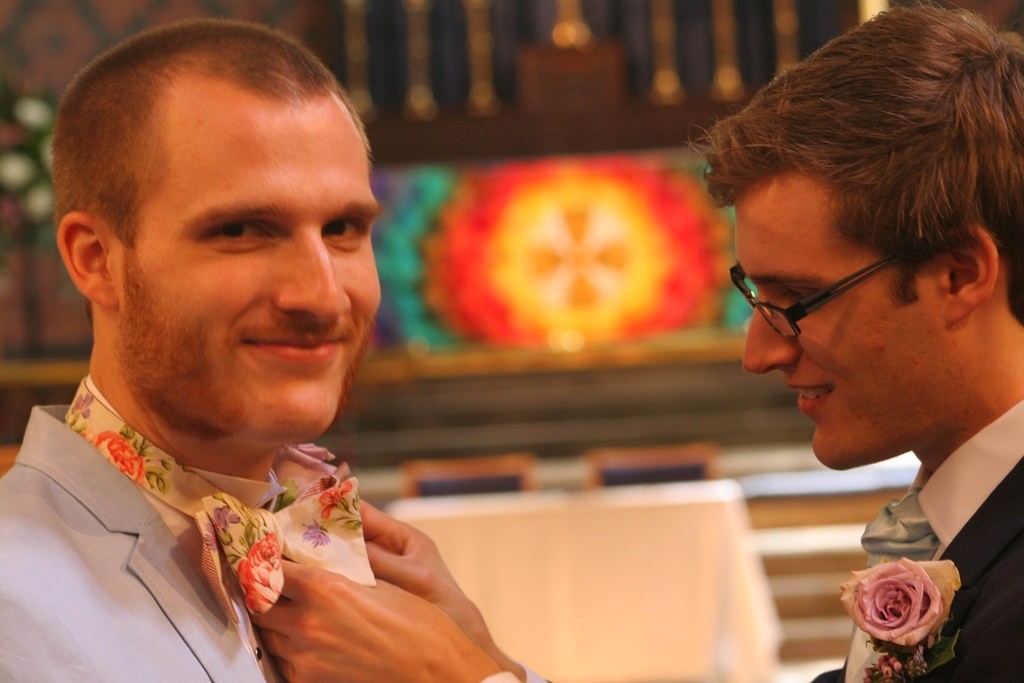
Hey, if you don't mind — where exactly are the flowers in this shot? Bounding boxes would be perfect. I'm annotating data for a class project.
[839,557,962,683]
[273,443,338,513]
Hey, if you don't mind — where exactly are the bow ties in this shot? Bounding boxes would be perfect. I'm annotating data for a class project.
[65,377,377,616]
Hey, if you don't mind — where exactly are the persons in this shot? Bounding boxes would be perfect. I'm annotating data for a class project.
[0,17,382,683]
[251,6,1024,683]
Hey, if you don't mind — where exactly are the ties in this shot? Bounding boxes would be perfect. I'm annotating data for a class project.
[844,493,940,683]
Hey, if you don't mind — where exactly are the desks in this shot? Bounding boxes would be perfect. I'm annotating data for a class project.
[382,477,787,683]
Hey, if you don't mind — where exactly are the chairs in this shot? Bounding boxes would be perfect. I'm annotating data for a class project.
[588,440,720,488]
[397,454,540,498]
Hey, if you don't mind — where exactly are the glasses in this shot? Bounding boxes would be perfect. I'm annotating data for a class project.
[729,256,903,336]
